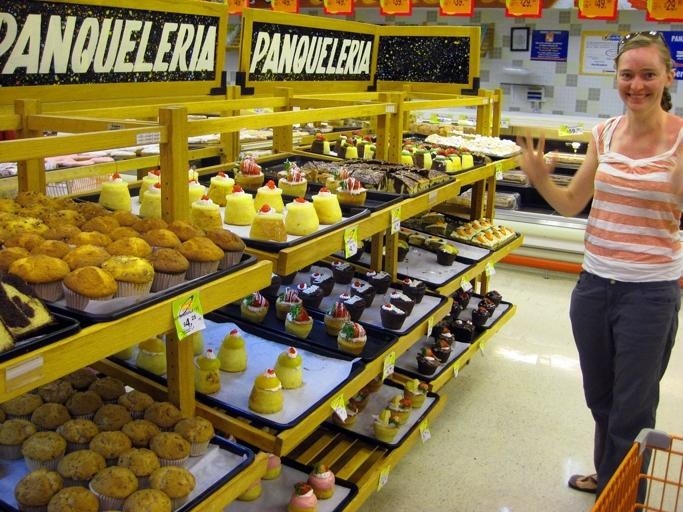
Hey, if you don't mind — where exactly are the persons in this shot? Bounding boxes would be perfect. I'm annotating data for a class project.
[510,25,683,512]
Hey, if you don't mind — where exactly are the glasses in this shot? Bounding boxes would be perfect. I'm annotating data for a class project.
[618,29,667,47]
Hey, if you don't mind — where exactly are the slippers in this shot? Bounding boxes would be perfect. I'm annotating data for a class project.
[567,473,599,494]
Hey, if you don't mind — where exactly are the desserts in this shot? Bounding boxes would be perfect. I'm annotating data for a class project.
[0,108,592,512]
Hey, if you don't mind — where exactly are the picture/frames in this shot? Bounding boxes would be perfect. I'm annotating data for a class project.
[509,25,529,54]
